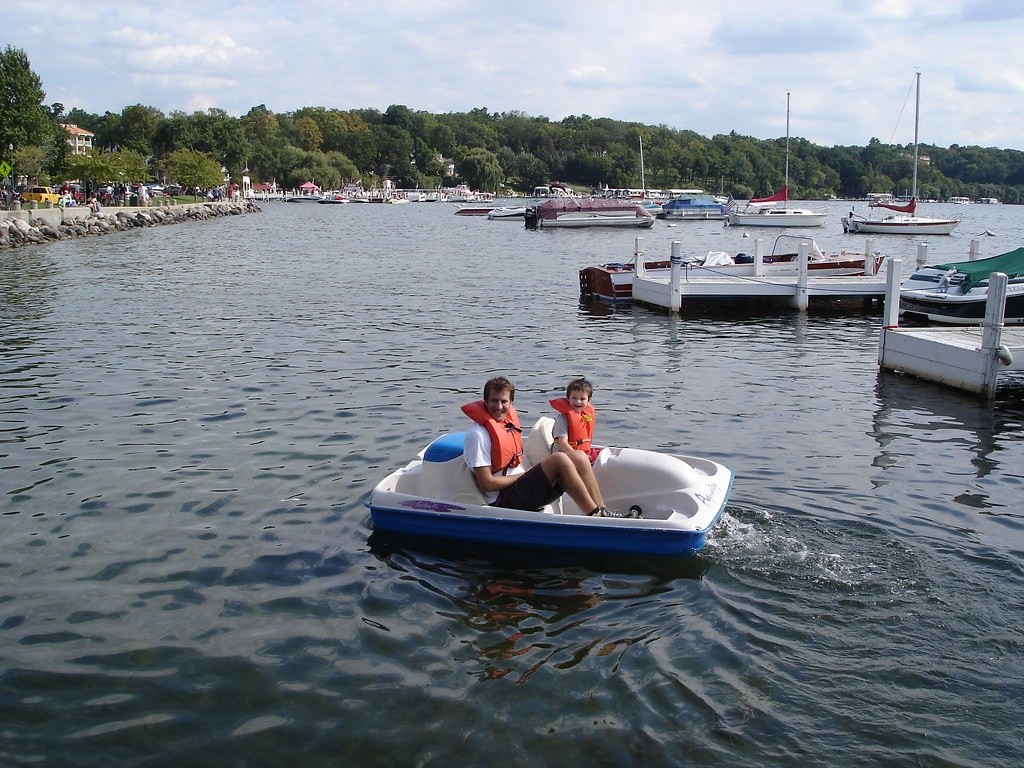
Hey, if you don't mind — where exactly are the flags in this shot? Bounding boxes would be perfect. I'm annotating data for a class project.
[724,196,736,213]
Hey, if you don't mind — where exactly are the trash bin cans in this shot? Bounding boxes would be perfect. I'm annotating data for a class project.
[130,196,139,206]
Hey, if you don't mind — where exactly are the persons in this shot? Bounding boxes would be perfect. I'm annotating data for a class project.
[59,179,75,207]
[9,193,26,208]
[550,378,596,465]
[106,184,122,207]
[228,187,233,202]
[85,194,102,214]
[206,185,224,203]
[138,182,147,206]
[463,376,644,519]
[330,192,341,200]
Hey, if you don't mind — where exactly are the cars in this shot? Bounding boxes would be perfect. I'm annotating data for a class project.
[20,183,86,203]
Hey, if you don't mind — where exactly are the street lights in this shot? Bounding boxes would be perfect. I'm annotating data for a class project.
[8,143,14,204]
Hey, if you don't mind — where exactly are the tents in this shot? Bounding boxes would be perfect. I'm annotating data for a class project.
[299,181,317,189]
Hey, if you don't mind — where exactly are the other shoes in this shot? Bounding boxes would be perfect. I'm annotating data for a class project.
[592,509,627,518]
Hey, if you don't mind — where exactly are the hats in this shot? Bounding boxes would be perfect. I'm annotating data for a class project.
[140,183,143,186]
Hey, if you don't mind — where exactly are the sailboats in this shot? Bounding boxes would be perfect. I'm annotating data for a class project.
[729,93,830,227]
[841,72,961,236]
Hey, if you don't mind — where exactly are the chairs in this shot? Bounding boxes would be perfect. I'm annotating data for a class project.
[526,417,556,469]
[453,462,488,507]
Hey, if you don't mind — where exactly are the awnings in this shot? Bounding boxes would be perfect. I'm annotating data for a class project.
[250,184,275,189]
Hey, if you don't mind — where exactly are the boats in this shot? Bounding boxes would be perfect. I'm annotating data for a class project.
[889,245,1024,324]
[286,192,493,205]
[662,194,729,222]
[524,198,656,228]
[364,433,735,560]
[454,184,583,221]
[578,235,890,307]
